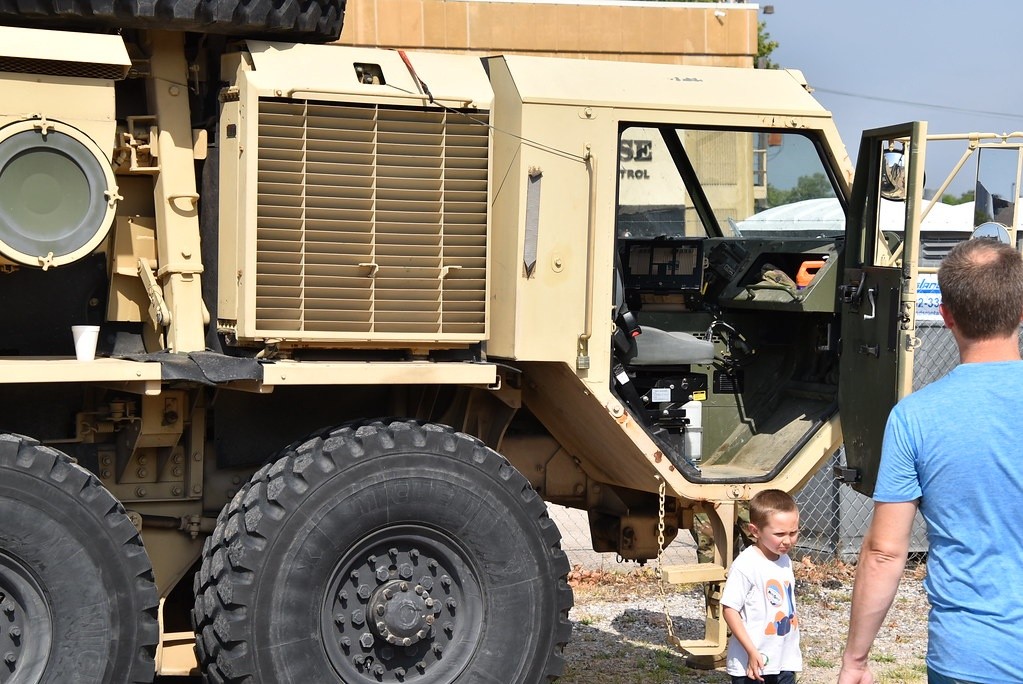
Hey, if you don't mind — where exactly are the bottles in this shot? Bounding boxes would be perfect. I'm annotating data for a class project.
[796,260,825,290]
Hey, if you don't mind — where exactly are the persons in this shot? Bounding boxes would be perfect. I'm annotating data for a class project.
[719,489,803,684]
[837,238,1023,684]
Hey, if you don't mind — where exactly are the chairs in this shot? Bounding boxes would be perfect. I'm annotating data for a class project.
[614,249,715,365]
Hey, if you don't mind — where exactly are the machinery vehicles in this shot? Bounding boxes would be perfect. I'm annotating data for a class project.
[0,0,1023,684]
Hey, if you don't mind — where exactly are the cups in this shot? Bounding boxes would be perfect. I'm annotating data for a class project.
[72,325,101,360]
